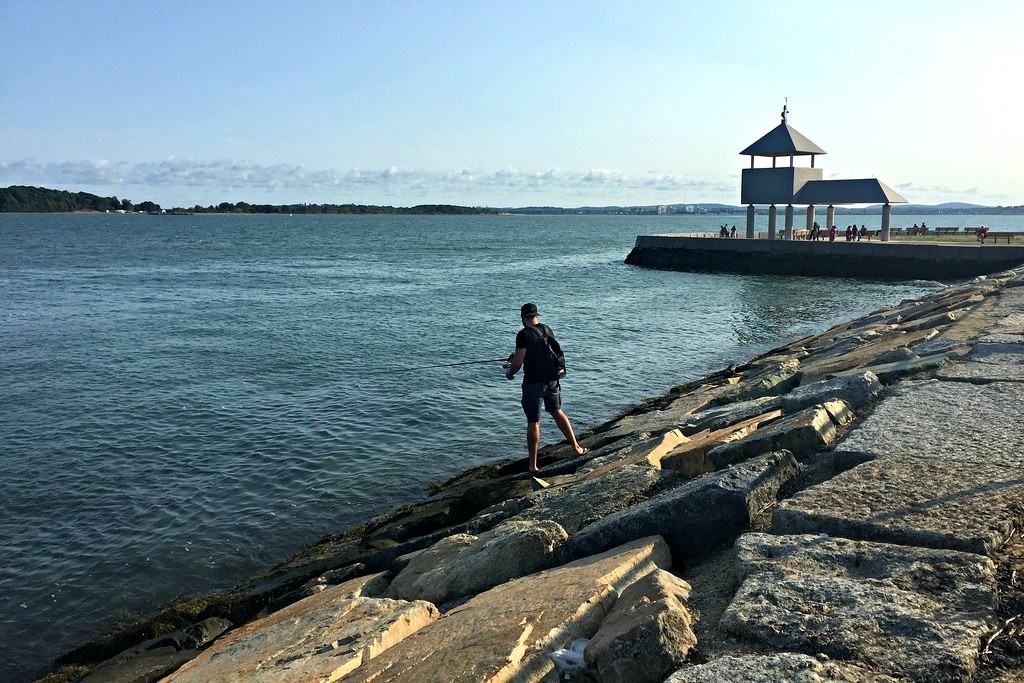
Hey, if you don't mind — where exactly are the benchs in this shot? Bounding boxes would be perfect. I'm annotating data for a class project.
[987,232,1014,244]
[890,228,902,235]
[858,231,875,241]
[816,228,829,240]
[905,228,929,235]
[964,227,989,235]
[779,230,810,240]
[935,227,959,235]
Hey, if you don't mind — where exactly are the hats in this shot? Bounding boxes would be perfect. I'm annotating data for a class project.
[521,303,539,316]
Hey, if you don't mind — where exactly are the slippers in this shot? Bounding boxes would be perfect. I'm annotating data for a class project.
[578,447,591,456]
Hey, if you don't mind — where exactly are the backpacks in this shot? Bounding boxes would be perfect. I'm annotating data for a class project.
[529,323,566,381]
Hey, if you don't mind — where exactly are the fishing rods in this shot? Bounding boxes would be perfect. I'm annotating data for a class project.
[394,352,516,372]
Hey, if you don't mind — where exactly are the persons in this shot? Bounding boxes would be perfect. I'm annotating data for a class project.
[981,226,986,241]
[914,224,918,227]
[809,222,820,241]
[846,225,867,241]
[505,303,591,471]
[922,223,925,227]
[829,226,836,241]
[720,224,736,237]
